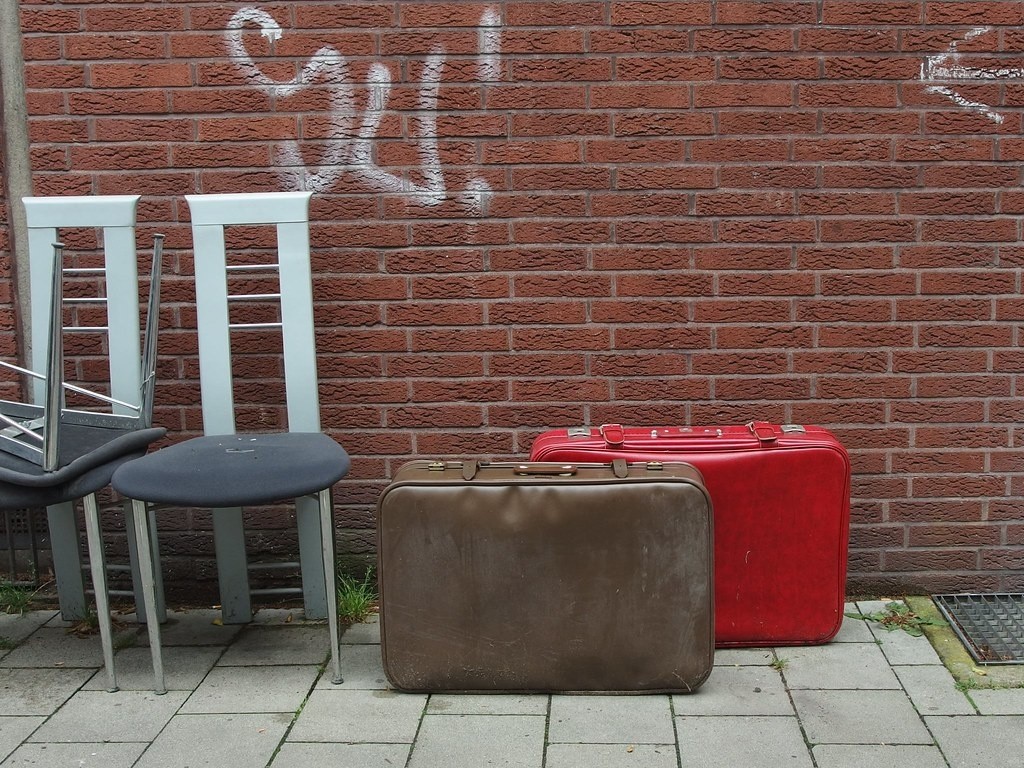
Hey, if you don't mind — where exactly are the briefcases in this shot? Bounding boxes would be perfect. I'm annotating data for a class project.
[377,459,715,696]
[528,421,849,648]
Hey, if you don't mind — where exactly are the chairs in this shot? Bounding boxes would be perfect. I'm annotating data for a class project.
[110,189,353,699]
[1,192,167,686]
